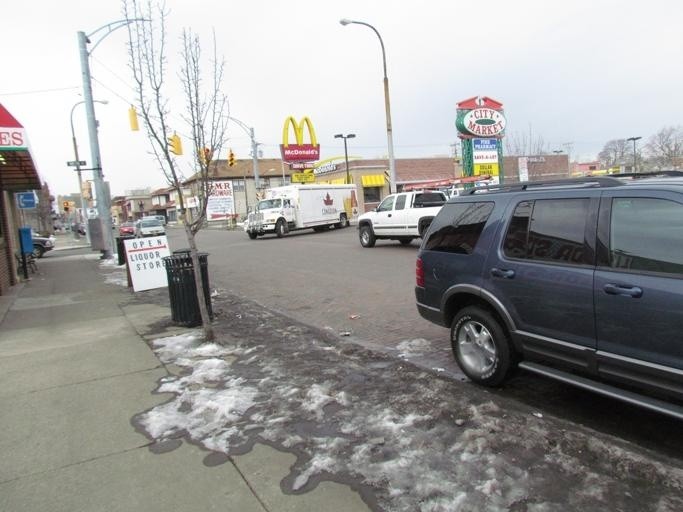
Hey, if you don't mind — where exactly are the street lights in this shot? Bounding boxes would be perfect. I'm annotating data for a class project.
[70,99,109,246]
[334,133,356,184]
[627,136,642,174]
[339,17,397,194]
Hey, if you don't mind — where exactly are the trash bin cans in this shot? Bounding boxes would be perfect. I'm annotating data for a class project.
[115,236,134,265]
[163,249,213,327]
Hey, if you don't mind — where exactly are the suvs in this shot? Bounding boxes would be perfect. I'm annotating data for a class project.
[414,170,682,422]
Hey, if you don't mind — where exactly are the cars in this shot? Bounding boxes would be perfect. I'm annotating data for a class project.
[119,215,167,238]
[31,236,55,258]
[70,221,87,235]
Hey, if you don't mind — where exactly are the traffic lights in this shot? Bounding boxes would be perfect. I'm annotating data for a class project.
[200,147,210,165]
[63,201,69,212]
[228,152,234,167]
[167,134,182,155]
[384,169,391,183]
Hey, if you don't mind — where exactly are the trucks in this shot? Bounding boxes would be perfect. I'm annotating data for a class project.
[246,183,359,239]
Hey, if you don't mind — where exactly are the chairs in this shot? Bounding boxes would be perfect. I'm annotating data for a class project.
[15,250,39,274]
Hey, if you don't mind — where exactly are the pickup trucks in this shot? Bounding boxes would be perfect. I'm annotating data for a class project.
[357,190,449,248]
